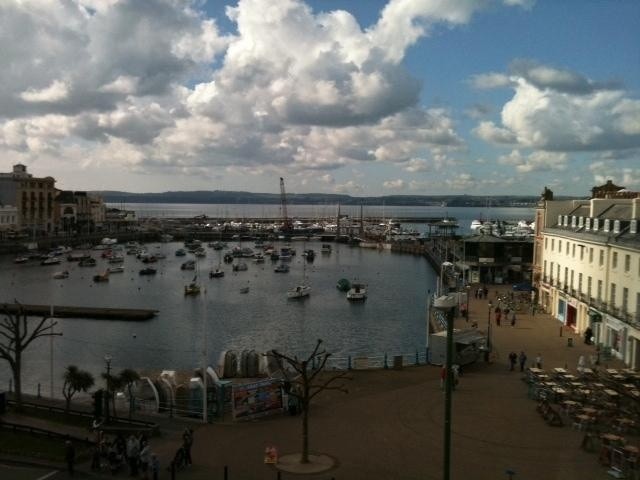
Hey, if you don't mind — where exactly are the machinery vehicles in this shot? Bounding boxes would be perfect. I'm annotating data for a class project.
[279,177,294,232]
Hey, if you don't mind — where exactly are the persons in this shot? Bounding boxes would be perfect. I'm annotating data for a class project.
[535,352,543,368]
[509,351,517,372]
[511,311,516,327]
[496,310,501,326]
[484,287,488,297]
[478,287,483,298]
[440,365,445,390]
[519,351,526,372]
[504,305,510,321]
[584,326,592,344]
[495,304,501,313]
[64,426,195,479]
[475,287,478,299]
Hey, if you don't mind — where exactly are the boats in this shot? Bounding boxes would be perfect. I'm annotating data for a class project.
[428,218,460,227]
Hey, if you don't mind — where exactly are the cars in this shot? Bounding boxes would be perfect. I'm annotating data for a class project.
[512,281,530,291]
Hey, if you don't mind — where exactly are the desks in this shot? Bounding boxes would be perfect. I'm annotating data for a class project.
[524,365,640,475]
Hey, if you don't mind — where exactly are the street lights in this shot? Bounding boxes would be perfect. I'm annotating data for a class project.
[466,286,470,322]
[104,352,112,426]
[484,304,491,361]
[434,295,458,479]
